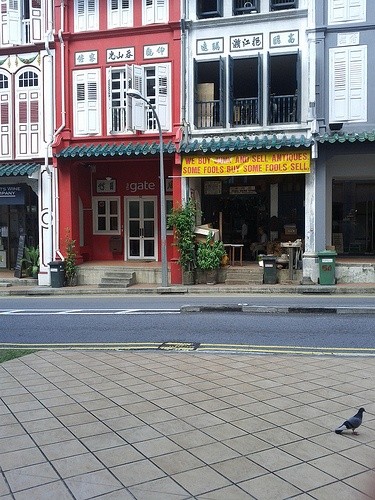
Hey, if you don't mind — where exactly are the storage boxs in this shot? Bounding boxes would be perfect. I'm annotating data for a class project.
[194,228,219,245]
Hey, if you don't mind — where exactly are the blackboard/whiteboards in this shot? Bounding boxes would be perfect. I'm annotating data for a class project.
[14,233,33,278]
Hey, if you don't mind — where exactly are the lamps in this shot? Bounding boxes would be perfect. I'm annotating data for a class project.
[329,123,343,130]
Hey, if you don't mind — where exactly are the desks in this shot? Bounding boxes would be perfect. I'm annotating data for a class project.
[224,244,244,266]
[282,247,300,280]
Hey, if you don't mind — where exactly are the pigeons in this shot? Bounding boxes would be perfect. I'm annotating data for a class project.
[335,407,367,436]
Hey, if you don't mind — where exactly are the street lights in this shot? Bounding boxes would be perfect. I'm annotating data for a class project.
[125,88,168,286]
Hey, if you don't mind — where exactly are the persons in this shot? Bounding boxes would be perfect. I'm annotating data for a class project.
[247,227,268,260]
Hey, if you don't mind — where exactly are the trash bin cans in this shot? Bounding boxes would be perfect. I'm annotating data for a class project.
[47,261,67,287]
[262,256,279,284]
[316,250,338,285]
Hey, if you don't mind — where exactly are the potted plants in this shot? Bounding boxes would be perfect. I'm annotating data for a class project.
[166,197,228,285]
[64,240,80,286]
[19,245,39,278]
